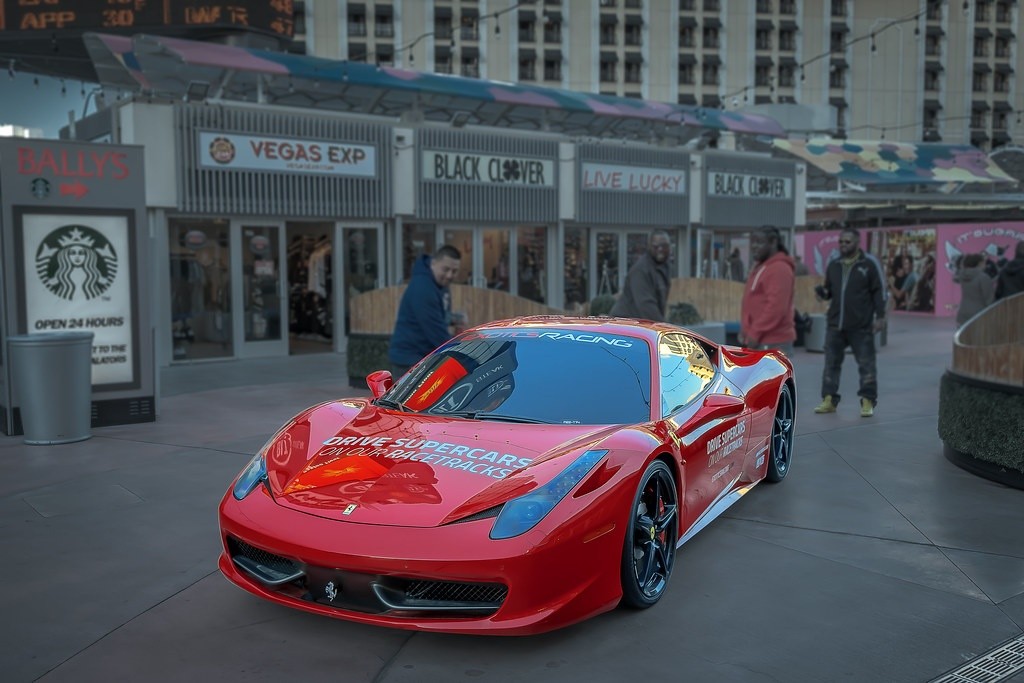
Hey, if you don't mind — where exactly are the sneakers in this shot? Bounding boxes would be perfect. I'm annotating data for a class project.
[861,397,874,417]
[814,394,837,413]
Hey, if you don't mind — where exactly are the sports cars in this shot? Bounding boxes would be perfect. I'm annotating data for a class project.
[217,315,798,638]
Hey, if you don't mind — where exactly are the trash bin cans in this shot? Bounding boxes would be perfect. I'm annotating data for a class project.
[725,322,747,348]
[6,331,95,446]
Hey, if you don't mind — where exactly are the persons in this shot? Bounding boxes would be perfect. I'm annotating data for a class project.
[385,244,467,383]
[736,223,797,360]
[723,248,743,281]
[889,254,935,313]
[952,241,1024,323]
[813,226,889,415]
[611,225,672,323]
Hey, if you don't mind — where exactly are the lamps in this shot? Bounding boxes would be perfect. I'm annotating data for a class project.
[185,80,210,103]
[450,111,470,128]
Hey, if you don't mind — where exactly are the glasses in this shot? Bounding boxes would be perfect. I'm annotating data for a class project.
[839,240,851,244]
[653,243,669,250]
